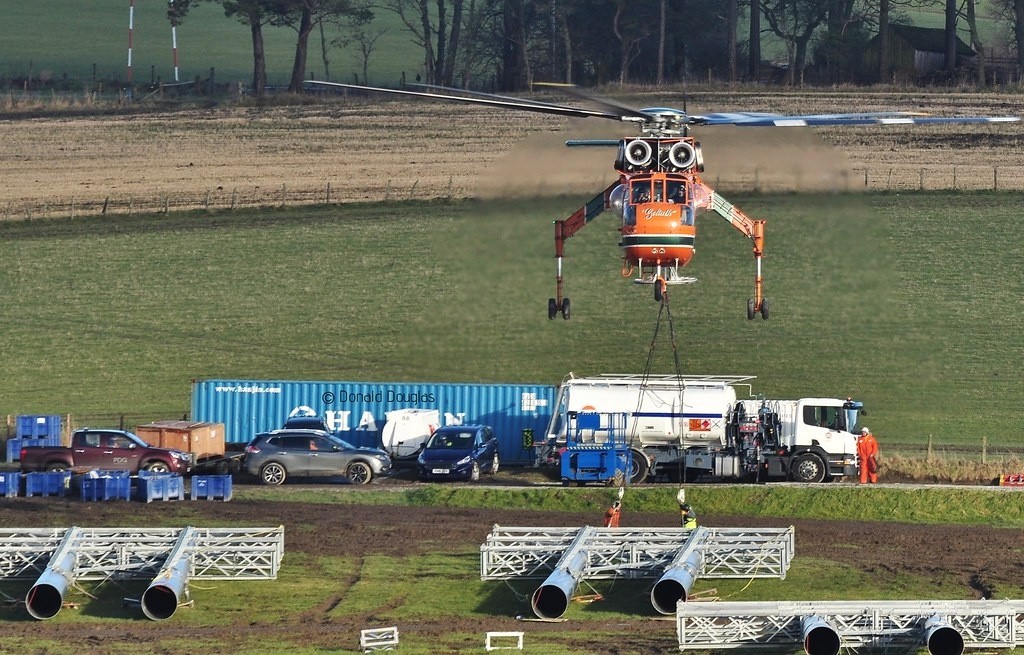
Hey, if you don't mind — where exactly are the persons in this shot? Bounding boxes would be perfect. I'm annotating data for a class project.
[856,427,879,485]
[680,504,697,527]
[633,187,650,203]
[668,185,686,204]
[603,501,623,527]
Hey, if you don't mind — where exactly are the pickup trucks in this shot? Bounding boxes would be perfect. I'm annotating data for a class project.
[20,427,192,477]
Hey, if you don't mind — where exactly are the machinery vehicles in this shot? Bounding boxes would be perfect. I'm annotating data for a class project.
[555,411,637,487]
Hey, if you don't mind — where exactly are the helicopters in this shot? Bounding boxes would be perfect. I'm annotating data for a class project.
[300,69,1024,323]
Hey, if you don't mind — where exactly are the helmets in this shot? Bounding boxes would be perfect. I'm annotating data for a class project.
[861,427,869,435]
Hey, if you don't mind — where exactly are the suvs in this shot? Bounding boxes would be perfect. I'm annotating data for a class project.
[416,426,500,483]
[282,415,333,434]
[242,430,392,486]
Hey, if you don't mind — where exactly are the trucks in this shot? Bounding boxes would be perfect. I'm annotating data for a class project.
[538,374,862,481]
